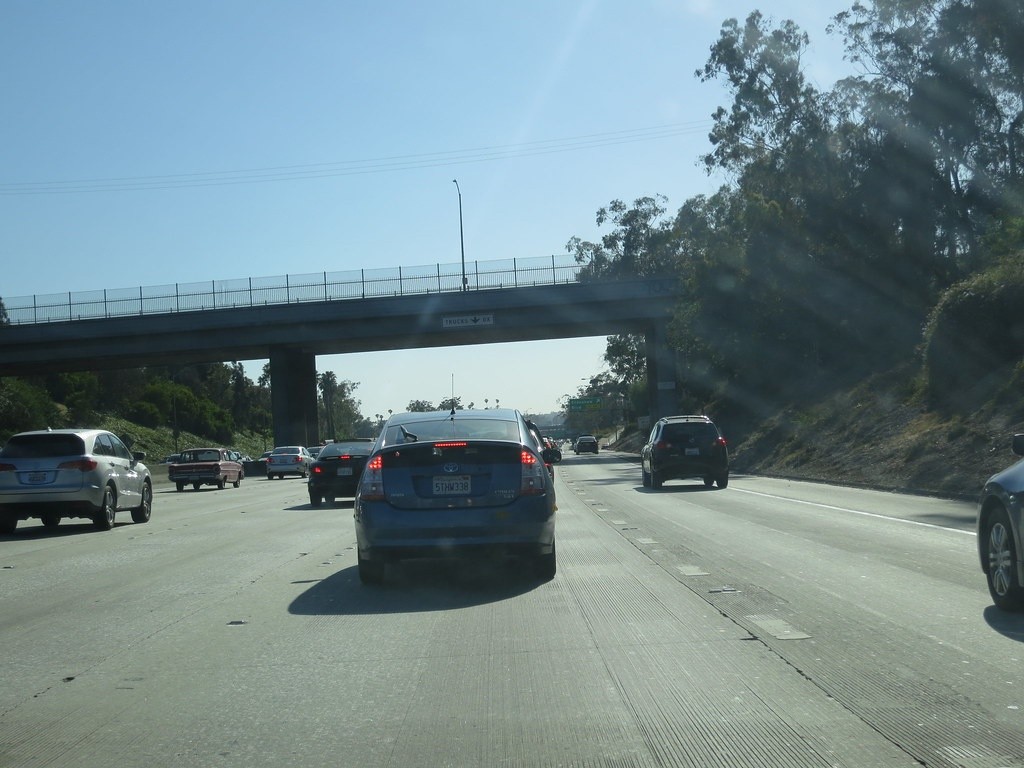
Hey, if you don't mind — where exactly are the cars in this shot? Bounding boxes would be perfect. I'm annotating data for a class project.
[169,448,243,491]
[309,441,380,506]
[307,446,326,461]
[266,446,316,480]
[574,435,600,455]
[167,454,192,465]
[1,425,153,533]
[258,451,274,463]
[352,407,562,585]
[977,433,1024,611]
[233,452,253,461]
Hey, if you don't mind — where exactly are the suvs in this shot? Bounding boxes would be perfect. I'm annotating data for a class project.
[640,414,729,490]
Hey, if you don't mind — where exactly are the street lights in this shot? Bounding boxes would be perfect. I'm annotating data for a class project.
[453,178,469,292]
